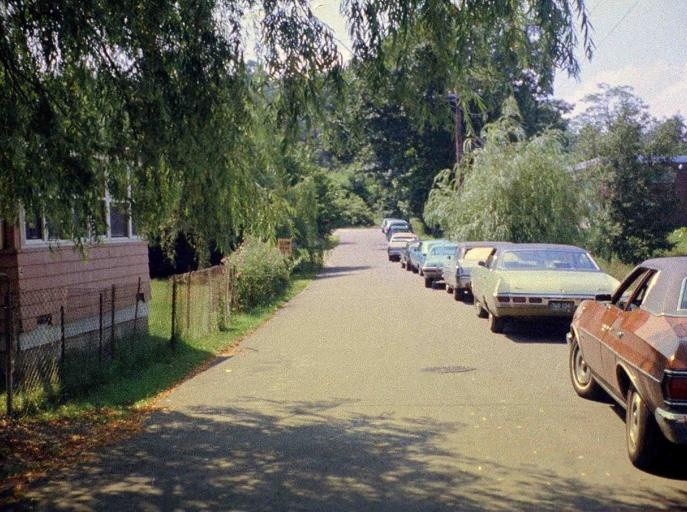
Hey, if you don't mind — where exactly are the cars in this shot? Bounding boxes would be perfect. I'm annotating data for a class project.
[566,256,687,469]
[381,217,622,334]
